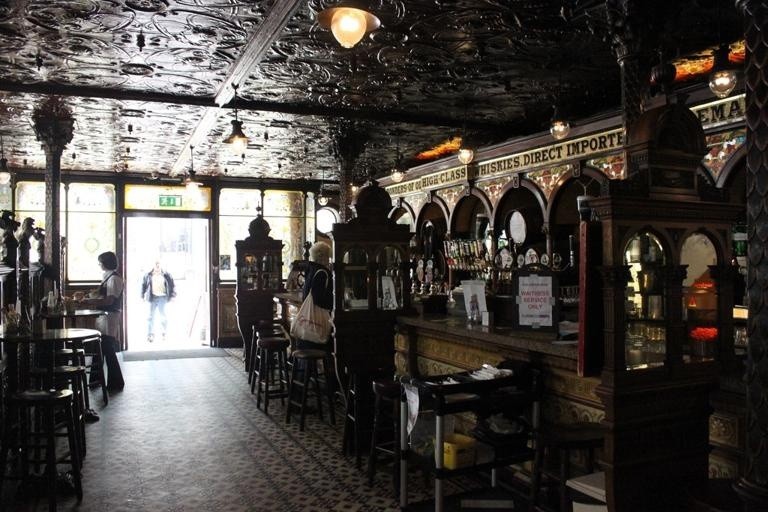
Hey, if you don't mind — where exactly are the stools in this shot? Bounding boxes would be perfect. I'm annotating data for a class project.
[528,420,607,509]
[10,387,85,511]
[247,321,401,494]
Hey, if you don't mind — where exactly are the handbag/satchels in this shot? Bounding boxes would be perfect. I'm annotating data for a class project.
[95,272,123,312]
[289,269,332,344]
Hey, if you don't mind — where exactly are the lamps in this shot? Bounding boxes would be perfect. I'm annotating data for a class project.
[704,13,742,101]
[317,1,385,49]
[390,128,406,182]
[222,79,252,156]
[548,70,571,141]
[315,167,330,207]
[456,95,474,165]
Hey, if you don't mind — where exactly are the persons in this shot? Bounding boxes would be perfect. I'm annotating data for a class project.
[299,242,333,345]
[140,261,177,342]
[80,251,125,391]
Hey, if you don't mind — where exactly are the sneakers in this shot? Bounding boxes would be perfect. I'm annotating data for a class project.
[289,401,319,414]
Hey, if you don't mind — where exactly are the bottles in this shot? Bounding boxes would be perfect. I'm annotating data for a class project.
[6,303,19,333]
[469,294,479,321]
[47,291,56,315]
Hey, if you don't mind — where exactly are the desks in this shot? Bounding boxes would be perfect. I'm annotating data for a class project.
[2,309,111,499]
